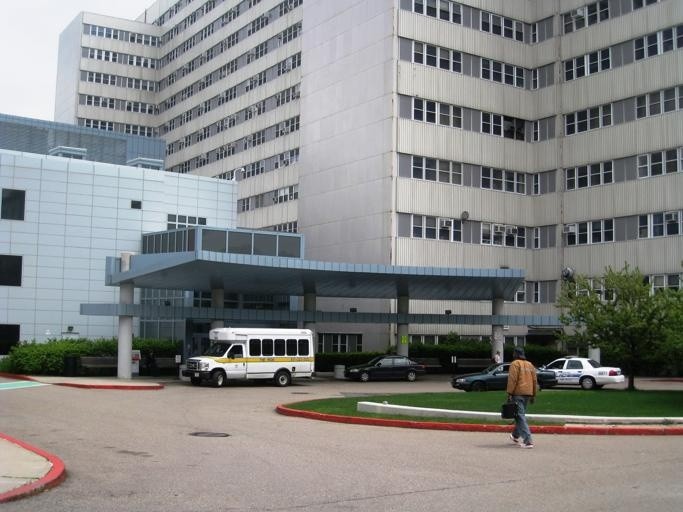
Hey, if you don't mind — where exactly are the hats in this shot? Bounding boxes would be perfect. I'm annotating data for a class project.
[514,347,526,360]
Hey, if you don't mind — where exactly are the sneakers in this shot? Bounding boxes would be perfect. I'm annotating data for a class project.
[510,434,519,442]
[520,443,533,447]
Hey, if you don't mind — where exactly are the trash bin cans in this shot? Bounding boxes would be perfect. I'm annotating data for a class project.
[131,350,141,377]
[64,356,81,377]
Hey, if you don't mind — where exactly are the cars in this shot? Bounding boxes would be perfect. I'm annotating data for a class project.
[343,354,426,383]
[539,356,626,391]
[451,361,556,394]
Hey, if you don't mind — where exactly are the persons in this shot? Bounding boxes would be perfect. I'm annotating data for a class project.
[490,350,504,374]
[504,346,539,450]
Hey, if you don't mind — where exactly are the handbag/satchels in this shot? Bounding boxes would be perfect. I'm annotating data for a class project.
[502,401,519,419]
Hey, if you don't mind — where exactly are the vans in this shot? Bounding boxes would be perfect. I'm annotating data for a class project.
[181,327,315,387]
